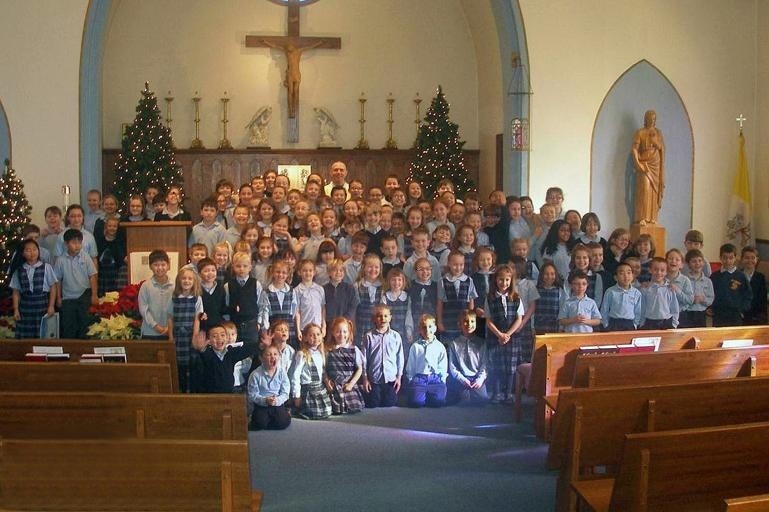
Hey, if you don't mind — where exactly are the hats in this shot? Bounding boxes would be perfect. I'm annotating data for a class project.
[685,230,703,242]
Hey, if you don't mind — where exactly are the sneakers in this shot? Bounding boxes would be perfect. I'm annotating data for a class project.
[502,393,513,404]
[490,392,504,404]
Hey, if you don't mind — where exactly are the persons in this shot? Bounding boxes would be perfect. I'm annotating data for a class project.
[196,324,275,415]
[471,246,496,336]
[144,185,159,221]
[84,188,104,233]
[137,249,175,339]
[634,234,656,281]
[272,320,295,373]
[252,237,276,285]
[408,258,438,334]
[484,263,525,404]
[53,204,98,270]
[625,257,641,287]
[419,201,432,221]
[222,252,263,356]
[38,205,63,257]
[361,303,405,407]
[247,344,291,430]
[396,207,424,264]
[365,205,385,252]
[381,213,391,232]
[402,226,441,282]
[53,229,100,339]
[483,204,499,227]
[368,187,392,207]
[274,174,290,190]
[531,262,566,336]
[289,322,333,420]
[8,239,58,339]
[520,196,544,232]
[682,249,714,328]
[448,309,488,408]
[534,204,556,267]
[304,178,321,212]
[340,199,360,227]
[589,243,613,291]
[563,244,602,308]
[272,186,291,217]
[153,185,191,221]
[215,191,236,228]
[681,229,712,277]
[565,210,581,231]
[224,205,249,246]
[507,197,542,252]
[153,193,167,214]
[429,224,452,268]
[239,183,254,206]
[282,248,301,288]
[314,107,336,139]
[94,194,123,235]
[348,179,364,200]
[7,224,50,274]
[263,259,297,340]
[331,185,346,222]
[706,244,751,327]
[323,316,364,414]
[640,248,693,327]
[541,219,574,286]
[180,243,208,278]
[96,215,128,292]
[316,194,333,217]
[390,212,407,237]
[235,241,251,258]
[251,106,272,136]
[168,266,204,392]
[243,224,261,255]
[438,179,463,204]
[404,180,424,211]
[249,196,261,220]
[338,215,362,259]
[192,313,253,387]
[285,189,303,221]
[632,109,666,225]
[425,197,455,241]
[307,173,324,185]
[212,241,231,285]
[436,251,478,343]
[256,198,277,238]
[342,231,369,281]
[557,272,602,333]
[443,191,456,207]
[289,200,310,237]
[456,225,478,276]
[740,246,768,325]
[391,189,408,220]
[602,228,632,273]
[507,255,540,375]
[322,209,340,241]
[465,213,494,251]
[313,240,336,283]
[406,314,450,407]
[251,176,265,197]
[512,237,539,285]
[293,260,327,341]
[216,178,235,208]
[379,267,414,342]
[600,263,641,331]
[322,259,359,343]
[385,175,400,201]
[465,193,480,213]
[270,213,298,261]
[324,160,352,201]
[383,204,392,214]
[199,258,225,327]
[545,187,566,221]
[574,212,606,249]
[187,198,225,256]
[275,213,335,266]
[489,190,507,207]
[353,253,383,344]
[380,235,403,276]
[118,193,151,289]
[263,170,278,197]
[450,204,466,250]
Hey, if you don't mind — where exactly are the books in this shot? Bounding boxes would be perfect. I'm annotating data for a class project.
[79,346,127,364]
[579,341,656,354]
[24,346,69,361]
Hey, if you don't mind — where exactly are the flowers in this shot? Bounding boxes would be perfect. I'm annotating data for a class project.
[84,280,148,340]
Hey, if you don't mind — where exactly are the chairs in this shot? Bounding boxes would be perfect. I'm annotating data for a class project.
[0,438,264,511]
[568,422,769,511]
[0,392,248,440]
[515,324,769,423]
[534,344,769,441]
[546,374,769,512]
[0,360,173,396]
[1,338,182,393]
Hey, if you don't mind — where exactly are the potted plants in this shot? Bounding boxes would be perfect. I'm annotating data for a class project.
[0,158,34,290]
[407,85,478,200]
[111,82,187,212]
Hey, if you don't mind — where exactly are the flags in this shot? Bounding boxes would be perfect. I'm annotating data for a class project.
[724,132,755,268]
